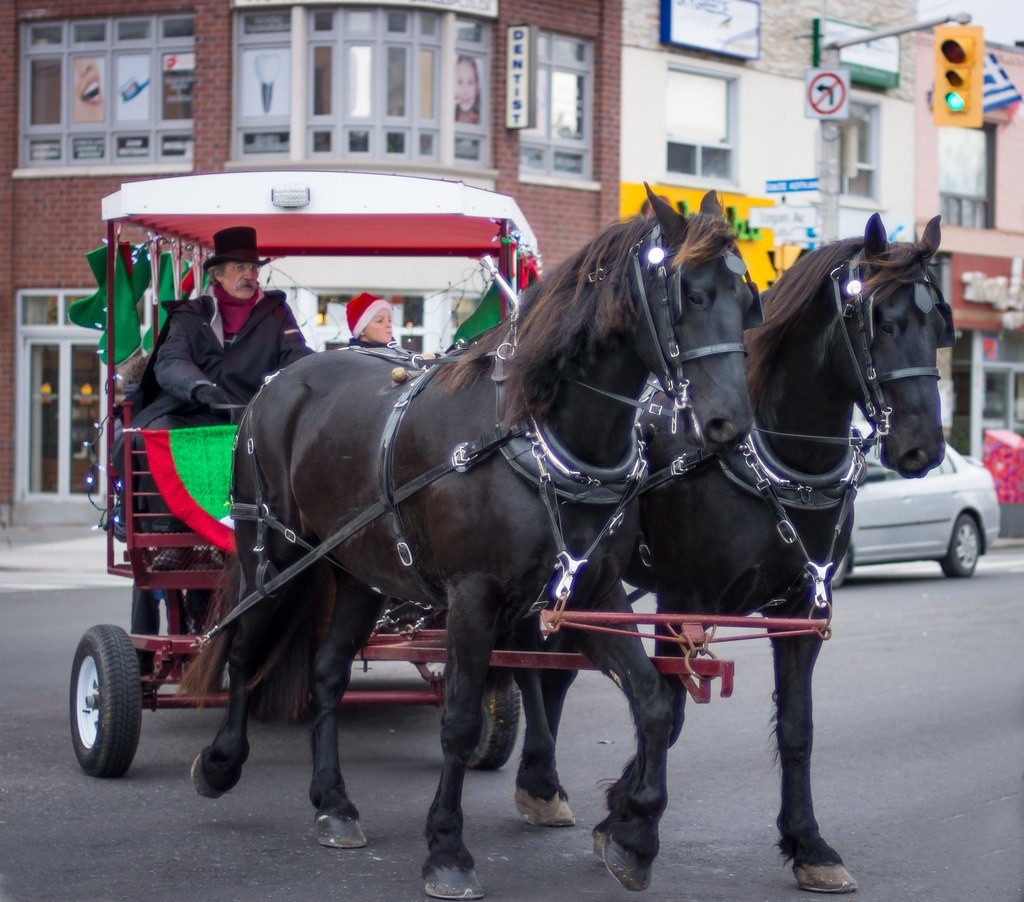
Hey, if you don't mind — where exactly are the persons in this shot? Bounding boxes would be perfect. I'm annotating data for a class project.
[454,56,480,124]
[110,357,163,674]
[108,225,319,569]
[347,292,400,349]
[70,56,105,123]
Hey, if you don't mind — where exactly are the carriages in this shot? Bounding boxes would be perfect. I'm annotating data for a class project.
[67,170,960,902]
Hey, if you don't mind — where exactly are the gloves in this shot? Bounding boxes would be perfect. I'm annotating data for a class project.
[193,385,228,414]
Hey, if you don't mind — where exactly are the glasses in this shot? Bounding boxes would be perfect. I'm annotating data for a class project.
[218,263,262,274]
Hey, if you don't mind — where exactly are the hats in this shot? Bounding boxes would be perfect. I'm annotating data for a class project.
[347,292,392,339]
[203,227,271,274]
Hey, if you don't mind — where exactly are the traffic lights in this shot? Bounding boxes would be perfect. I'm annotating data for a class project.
[933,24,985,130]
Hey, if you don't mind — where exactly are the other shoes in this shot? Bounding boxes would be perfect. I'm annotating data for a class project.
[152,545,192,570]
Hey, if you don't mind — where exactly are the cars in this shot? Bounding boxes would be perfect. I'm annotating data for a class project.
[827,421,1004,590]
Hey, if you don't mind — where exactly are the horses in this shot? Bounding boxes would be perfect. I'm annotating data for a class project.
[504,212,956,896]
[188,181,762,901]
